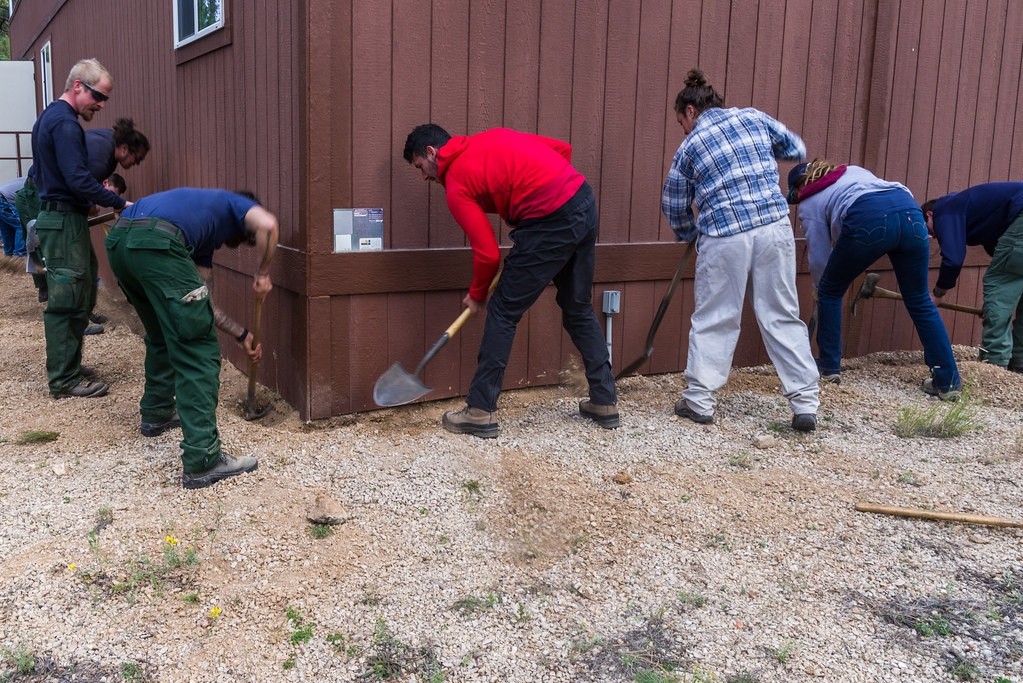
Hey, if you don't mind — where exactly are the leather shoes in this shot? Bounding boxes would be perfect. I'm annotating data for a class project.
[183,449,258,488]
[141,410,183,437]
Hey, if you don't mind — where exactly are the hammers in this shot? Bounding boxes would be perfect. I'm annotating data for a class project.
[852,272,983,316]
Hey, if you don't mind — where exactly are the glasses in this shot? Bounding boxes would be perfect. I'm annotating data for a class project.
[78,81,110,103]
[130,149,141,165]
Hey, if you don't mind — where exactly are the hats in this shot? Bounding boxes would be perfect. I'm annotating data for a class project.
[786,162,808,204]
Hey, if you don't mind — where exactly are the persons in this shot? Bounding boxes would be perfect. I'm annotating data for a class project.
[660,69,819,432]
[103,187,279,489]
[785,162,963,402]
[31,58,135,399]
[919,182,1023,372]
[0,119,150,336]
[402,123,619,439]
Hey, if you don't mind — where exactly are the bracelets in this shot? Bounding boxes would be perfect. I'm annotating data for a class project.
[235,328,249,343]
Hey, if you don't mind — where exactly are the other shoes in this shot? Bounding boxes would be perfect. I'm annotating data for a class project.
[90,313,108,324]
[922,378,962,402]
[815,360,842,386]
[791,413,816,430]
[83,323,104,335]
[675,399,713,422]
[52,377,109,400]
[79,364,101,380]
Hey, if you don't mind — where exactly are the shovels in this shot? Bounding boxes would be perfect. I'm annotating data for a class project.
[96,213,137,317]
[373,269,503,407]
[614,236,697,382]
[242,228,270,421]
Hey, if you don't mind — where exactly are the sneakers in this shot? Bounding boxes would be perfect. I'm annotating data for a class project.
[579,398,620,429]
[441,405,499,439]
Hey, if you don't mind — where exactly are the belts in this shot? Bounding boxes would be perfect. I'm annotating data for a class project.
[40,199,84,213]
[115,217,186,245]
